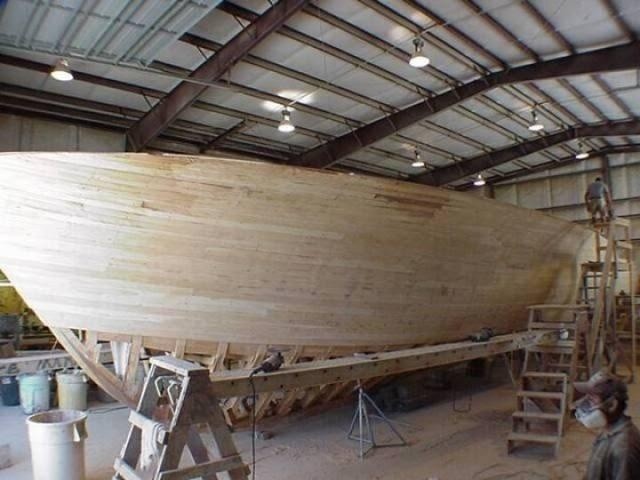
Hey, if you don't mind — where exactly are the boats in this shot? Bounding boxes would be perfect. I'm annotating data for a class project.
[1,150,632,433]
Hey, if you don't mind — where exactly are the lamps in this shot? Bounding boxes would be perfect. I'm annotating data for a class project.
[411,153,425,167]
[408,33,430,68]
[278,109,295,132]
[527,111,544,131]
[473,170,486,186]
[50,58,75,81]
[575,142,590,160]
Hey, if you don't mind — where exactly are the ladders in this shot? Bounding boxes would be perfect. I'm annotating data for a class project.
[109,355,250,480]
[505,304,591,458]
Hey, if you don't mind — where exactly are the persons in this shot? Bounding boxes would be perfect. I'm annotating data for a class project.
[584,177,612,223]
[569,368,640,480]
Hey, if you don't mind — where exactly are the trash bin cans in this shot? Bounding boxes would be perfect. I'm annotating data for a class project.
[3,372,89,411]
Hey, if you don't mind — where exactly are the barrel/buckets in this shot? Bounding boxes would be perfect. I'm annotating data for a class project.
[18,372,53,414]
[55,369,89,409]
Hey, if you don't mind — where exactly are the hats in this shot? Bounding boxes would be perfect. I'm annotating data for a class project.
[572,370,628,401]
